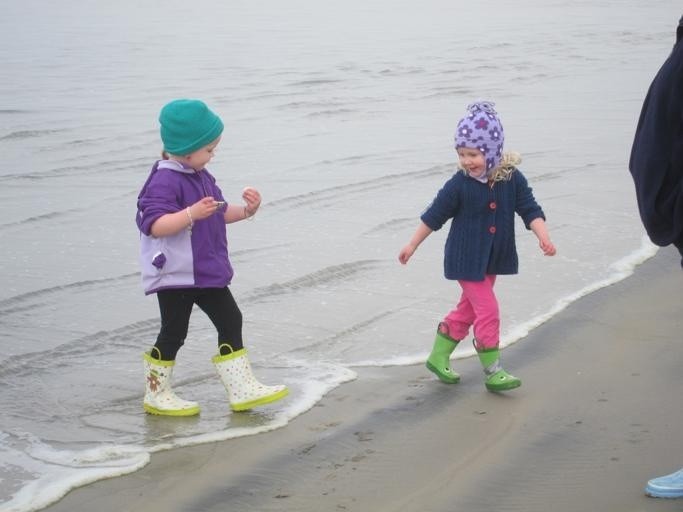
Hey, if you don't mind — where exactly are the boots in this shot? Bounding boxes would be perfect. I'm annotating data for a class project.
[426,323,460,383]
[473,337,521,391]
[212,344,289,412]
[143,346,200,416]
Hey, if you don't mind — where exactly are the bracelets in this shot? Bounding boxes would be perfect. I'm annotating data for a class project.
[244,207,255,220]
[184,205,194,229]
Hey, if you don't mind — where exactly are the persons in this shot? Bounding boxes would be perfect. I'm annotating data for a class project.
[399,99,557,390]
[132,98,289,418]
[627,16,683,500]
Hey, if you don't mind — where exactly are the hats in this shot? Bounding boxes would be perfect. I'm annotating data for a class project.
[159,99,224,156]
[455,102,504,179]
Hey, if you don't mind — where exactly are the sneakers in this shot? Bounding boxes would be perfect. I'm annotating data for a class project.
[645,469,683,498]
